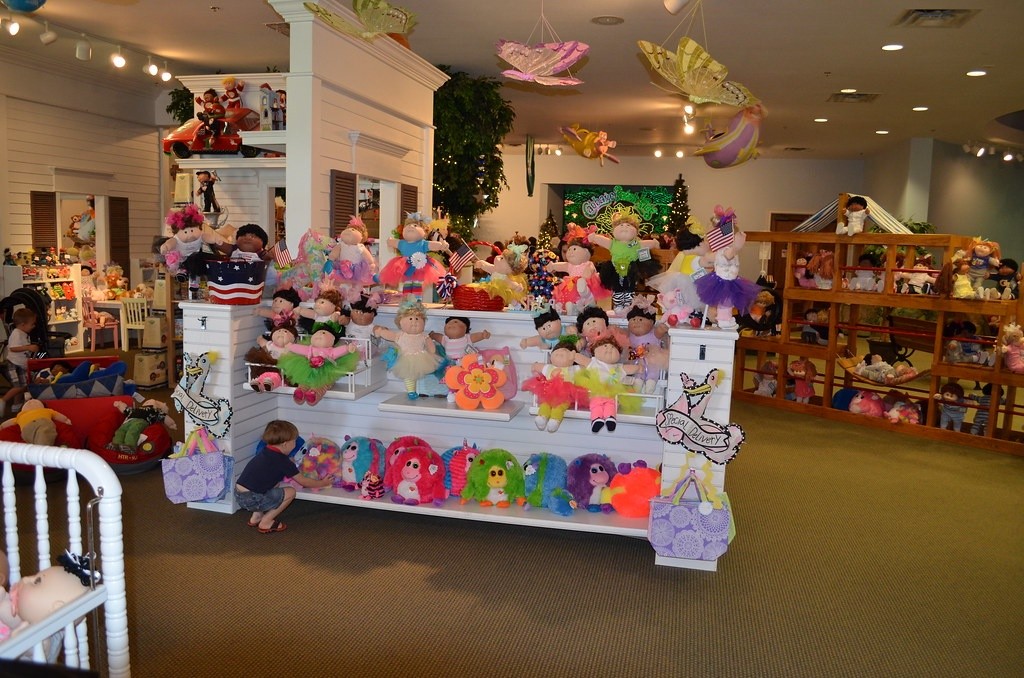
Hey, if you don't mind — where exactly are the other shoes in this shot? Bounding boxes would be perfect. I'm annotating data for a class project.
[0,399,7,418]
[12,404,22,412]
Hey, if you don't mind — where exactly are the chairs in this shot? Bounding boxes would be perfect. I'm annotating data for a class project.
[121,299,147,351]
[82,297,119,352]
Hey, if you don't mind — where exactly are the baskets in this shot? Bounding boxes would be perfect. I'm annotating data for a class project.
[449,242,507,310]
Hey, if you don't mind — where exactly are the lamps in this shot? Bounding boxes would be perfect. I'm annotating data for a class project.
[545,144,551,155]
[143,56,158,76]
[110,45,126,68]
[962,140,1024,162]
[555,144,563,156]
[158,60,172,82]
[2,12,20,35]
[538,144,543,155]
[75,33,93,61]
[40,20,59,45]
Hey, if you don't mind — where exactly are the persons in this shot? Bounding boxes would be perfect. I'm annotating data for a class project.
[0,548,101,664]
[0,308,39,419]
[234,419,336,533]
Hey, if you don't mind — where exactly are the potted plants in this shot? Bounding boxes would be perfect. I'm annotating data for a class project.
[859,217,937,365]
[433,64,517,285]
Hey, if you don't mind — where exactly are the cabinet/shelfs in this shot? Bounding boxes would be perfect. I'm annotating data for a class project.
[166,270,183,388]
[3,264,83,354]
[176,296,739,572]
[731,193,1024,457]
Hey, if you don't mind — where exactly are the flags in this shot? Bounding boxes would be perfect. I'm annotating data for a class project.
[274,238,292,269]
[707,222,734,253]
[449,243,475,273]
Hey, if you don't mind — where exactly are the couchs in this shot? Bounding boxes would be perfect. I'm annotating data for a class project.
[0,355,171,485]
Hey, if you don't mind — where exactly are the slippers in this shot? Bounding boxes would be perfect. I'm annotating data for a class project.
[247,517,288,534]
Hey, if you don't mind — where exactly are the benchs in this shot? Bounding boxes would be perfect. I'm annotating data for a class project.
[887,315,999,390]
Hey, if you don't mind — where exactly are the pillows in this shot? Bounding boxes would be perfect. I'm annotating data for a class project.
[28,383,76,399]
[75,373,123,398]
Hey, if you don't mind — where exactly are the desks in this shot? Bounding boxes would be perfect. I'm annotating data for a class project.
[84,299,154,349]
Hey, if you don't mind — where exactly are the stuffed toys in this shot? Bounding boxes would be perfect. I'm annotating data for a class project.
[80,263,154,327]
[60,197,96,268]
[748,196,1024,437]
[0,360,177,476]
[256,432,663,518]
[152,205,776,329]
[195,77,245,136]
[3,246,79,346]
[196,170,221,213]
[243,286,669,433]
